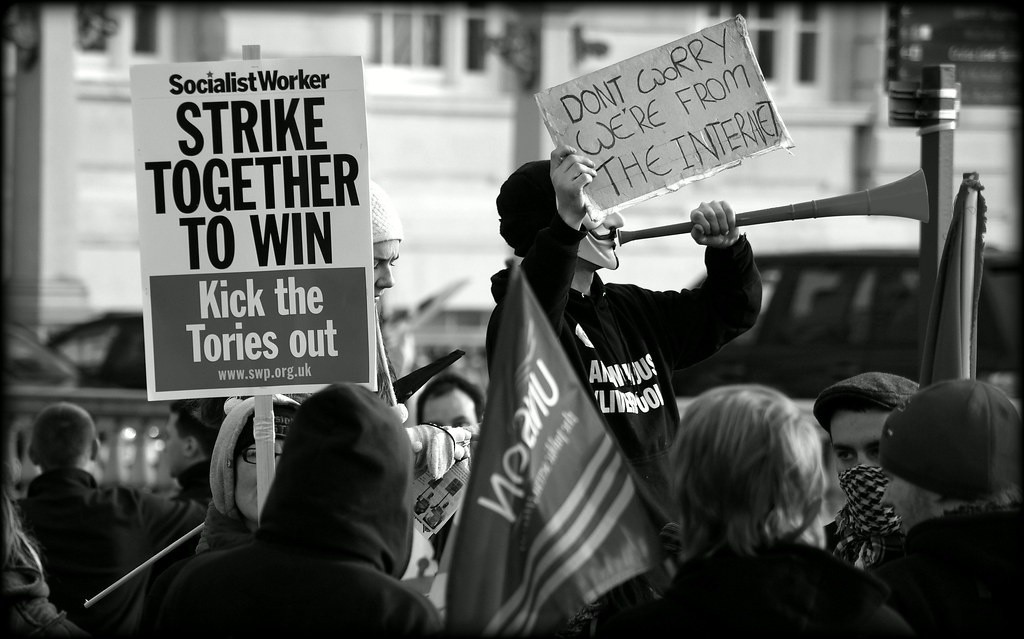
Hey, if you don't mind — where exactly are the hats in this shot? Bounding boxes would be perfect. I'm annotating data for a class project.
[234,404,298,456]
[813,372,920,431]
[878,378,1023,499]
[496,160,550,256]
[369,179,403,244]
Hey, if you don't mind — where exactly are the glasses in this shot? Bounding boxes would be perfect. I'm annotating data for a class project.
[241,447,280,465]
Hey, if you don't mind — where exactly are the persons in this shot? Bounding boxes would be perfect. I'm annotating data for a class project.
[0,144,1024,639]
